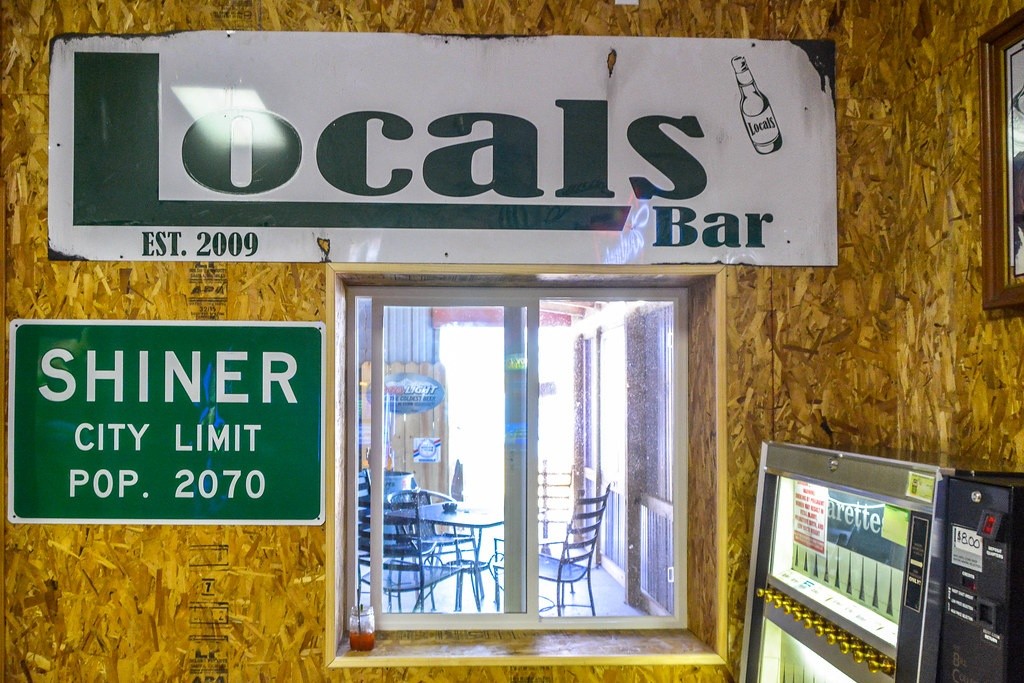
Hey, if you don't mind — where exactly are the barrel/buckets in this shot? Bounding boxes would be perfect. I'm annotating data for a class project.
[384,472,414,503]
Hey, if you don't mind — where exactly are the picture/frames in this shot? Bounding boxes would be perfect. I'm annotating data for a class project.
[977,8,1024,311]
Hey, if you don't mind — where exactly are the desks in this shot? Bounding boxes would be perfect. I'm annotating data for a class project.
[389,500,547,613]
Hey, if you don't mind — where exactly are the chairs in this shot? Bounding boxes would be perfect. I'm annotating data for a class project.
[496,465,615,617]
[359,469,486,613]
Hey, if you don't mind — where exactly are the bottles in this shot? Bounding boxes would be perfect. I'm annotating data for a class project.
[349,606,375,651]
